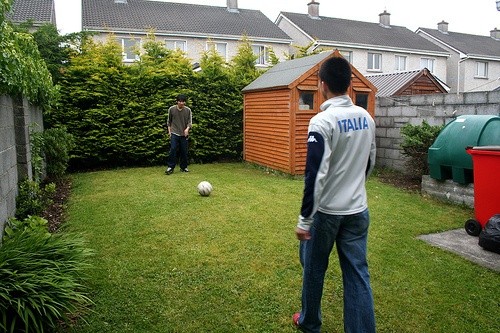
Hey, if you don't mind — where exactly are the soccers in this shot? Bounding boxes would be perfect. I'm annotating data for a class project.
[198,180,213,197]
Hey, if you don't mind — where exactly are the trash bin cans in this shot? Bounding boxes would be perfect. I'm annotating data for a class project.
[465,145,500,237]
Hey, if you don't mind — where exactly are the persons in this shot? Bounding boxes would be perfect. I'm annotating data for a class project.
[293,57,376,333]
[165,95,192,175]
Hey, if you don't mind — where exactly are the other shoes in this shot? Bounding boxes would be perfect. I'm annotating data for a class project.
[180,168,189,172]
[165,167,174,175]
[293,312,300,326]
